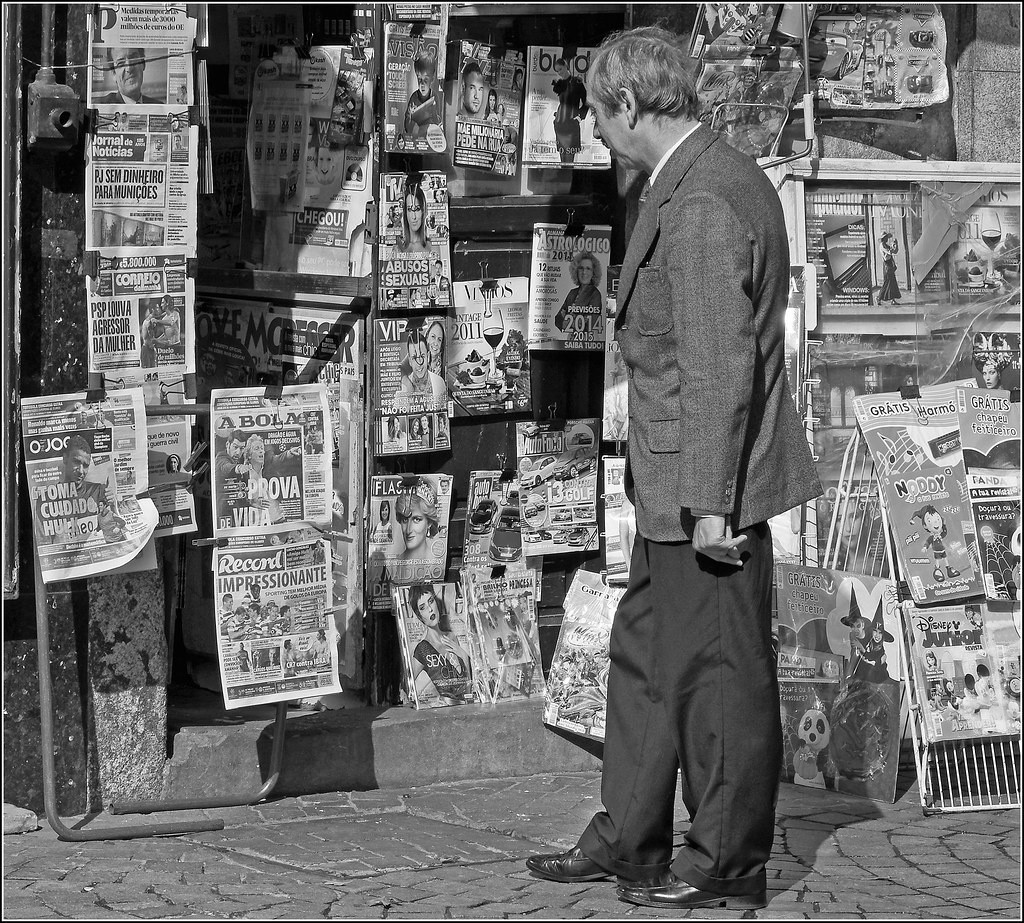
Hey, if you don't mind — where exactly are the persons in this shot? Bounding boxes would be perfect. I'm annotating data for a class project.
[848,617,889,683]
[174,136,188,151]
[216,426,322,528]
[176,86,187,104]
[36,436,125,547]
[980,362,1003,389]
[220,584,328,677]
[403,50,590,168]
[373,476,471,707]
[875,231,902,305]
[96,48,164,104]
[524,26,825,909]
[385,321,449,449]
[109,112,128,131]
[385,186,450,309]
[141,294,181,369]
[166,454,181,474]
[555,251,601,340]
[617,497,636,570]
[315,147,340,185]
[836,497,881,576]
[605,352,629,440]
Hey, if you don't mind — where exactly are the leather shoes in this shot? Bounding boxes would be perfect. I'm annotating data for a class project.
[526,845,617,883]
[616,868,767,909]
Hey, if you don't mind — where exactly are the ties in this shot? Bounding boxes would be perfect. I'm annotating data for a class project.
[638,179,652,216]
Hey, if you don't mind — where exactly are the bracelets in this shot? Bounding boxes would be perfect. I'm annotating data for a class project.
[620,429,626,434]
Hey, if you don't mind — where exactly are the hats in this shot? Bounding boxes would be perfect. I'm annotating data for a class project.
[880,231,892,239]
[841,582,871,629]
[904,375,912,382]
[872,594,894,643]
[972,332,1014,372]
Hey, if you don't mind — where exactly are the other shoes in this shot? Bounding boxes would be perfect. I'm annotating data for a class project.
[891,300,899,305]
[875,295,882,305]
